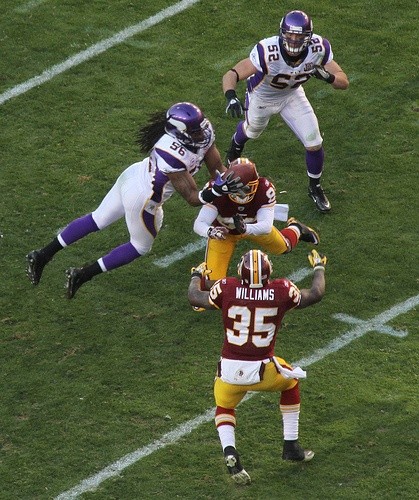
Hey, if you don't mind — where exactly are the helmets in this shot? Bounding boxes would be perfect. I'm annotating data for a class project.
[223,157,259,204]
[237,249,273,288]
[280,10,313,57]
[164,102,211,148]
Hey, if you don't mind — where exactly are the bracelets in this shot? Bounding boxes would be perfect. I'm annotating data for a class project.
[314,266,325,271]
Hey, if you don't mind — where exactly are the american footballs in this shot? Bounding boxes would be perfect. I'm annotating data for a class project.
[222,164,254,191]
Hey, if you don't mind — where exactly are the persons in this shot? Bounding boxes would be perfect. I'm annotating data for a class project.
[192,157,319,312]
[188,250,327,484]
[26,102,243,300]
[222,11,349,212]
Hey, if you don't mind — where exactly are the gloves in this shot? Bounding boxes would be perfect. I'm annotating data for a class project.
[308,249,327,271]
[207,226,229,240]
[212,172,243,197]
[191,262,212,279]
[232,213,247,234]
[309,65,335,84]
[225,89,248,119]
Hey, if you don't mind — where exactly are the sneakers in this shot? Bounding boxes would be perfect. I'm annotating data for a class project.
[64,266,85,299]
[225,135,244,169]
[192,306,206,312]
[25,250,45,285]
[224,454,252,486]
[287,217,320,246]
[282,450,315,461]
[308,184,332,213]
